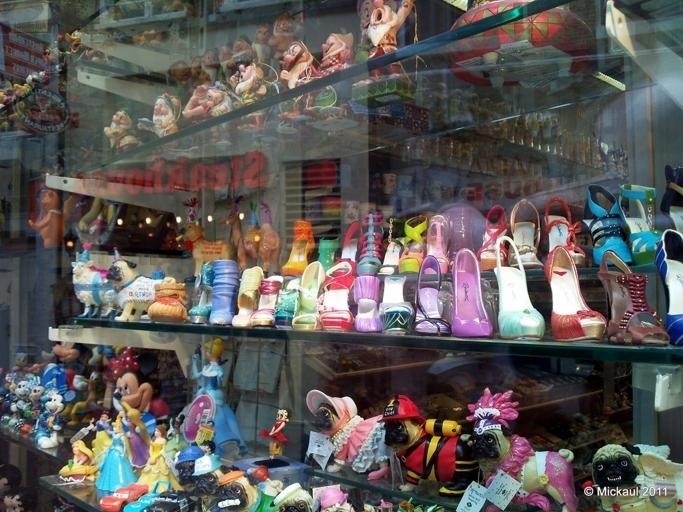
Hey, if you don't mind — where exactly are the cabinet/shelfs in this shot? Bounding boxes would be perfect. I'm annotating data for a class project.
[45,1,682,510]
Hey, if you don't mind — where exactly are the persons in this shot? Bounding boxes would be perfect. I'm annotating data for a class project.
[258,409,291,456]
[60,338,248,495]
[104,1,414,157]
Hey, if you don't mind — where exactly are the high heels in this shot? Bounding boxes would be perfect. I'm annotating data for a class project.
[596,250,669,345]
[544,246,606,340]
[232,214,451,335]
[187,263,214,325]
[660,165,682,230]
[477,205,507,269]
[493,236,545,339]
[451,249,493,339]
[581,185,635,266]
[542,197,586,266]
[507,198,544,267]
[657,229,682,346]
[618,185,659,263]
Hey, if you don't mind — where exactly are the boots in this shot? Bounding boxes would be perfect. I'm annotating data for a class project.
[210,260,238,327]
[148,284,186,322]
